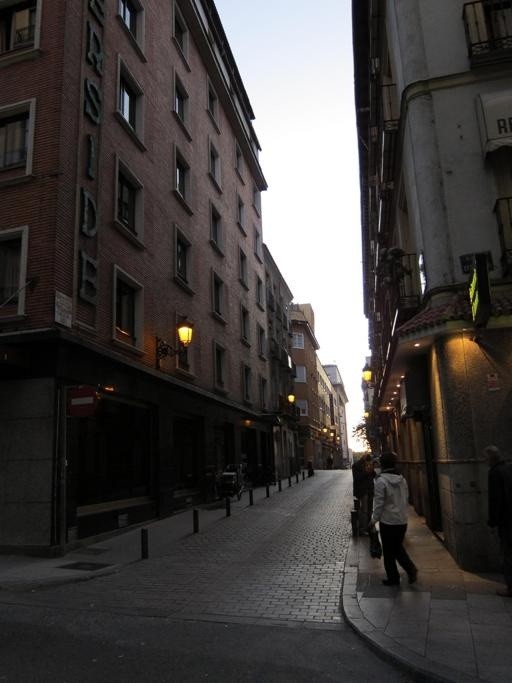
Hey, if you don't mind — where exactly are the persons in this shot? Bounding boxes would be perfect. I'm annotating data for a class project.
[482,444,512,597]
[352,451,417,586]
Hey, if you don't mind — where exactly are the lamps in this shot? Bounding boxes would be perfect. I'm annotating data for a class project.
[361,363,376,390]
[323,425,341,443]
[277,391,295,412]
[157,314,194,362]
